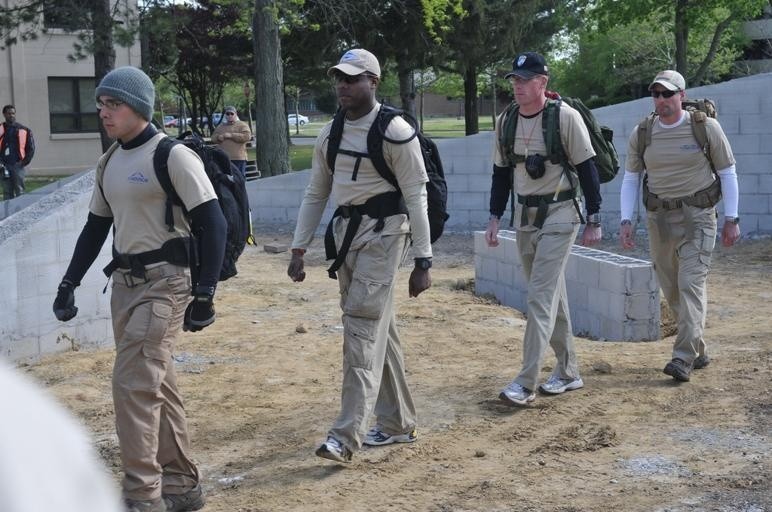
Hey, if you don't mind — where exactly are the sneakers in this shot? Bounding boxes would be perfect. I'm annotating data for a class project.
[538,375,584,395]
[315,436,353,463]
[124,495,168,512]
[161,482,206,512]
[363,424,418,446]
[663,358,695,381]
[694,354,710,369]
[498,382,536,405]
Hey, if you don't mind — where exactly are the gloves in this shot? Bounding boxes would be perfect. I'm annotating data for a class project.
[52,280,78,321]
[183,294,217,332]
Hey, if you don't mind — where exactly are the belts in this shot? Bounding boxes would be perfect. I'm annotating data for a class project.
[111,263,183,287]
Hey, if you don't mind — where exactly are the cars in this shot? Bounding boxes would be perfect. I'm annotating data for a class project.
[164,113,228,128]
[287,114,309,127]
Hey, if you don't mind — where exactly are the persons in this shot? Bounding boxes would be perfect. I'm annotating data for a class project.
[0,104,35,200]
[209,104,252,179]
[50,64,229,511]
[619,68,740,382]
[481,48,605,407]
[284,47,436,463]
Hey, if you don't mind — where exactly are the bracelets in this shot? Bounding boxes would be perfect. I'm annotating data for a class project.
[620,219,632,225]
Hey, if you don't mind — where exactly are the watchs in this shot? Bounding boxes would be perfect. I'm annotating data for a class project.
[585,213,602,223]
[414,258,433,269]
[725,215,739,225]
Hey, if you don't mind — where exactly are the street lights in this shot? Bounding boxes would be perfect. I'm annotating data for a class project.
[243,81,253,133]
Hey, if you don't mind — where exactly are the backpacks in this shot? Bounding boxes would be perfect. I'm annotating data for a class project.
[498,96,620,230]
[324,101,450,279]
[154,130,251,283]
[637,98,721,243]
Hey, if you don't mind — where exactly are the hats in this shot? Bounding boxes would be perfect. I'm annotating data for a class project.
[648,70,685,92]
[94,66,156,123]
[503,52,548,79]
[225,106,236,113]
[327,49,381,80]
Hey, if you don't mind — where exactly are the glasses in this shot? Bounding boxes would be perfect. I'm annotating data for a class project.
[508,76,537,85]
[226,112,235,116]
[94,99,123,111]
[651,89,675,98]
[333,71,376,84]
